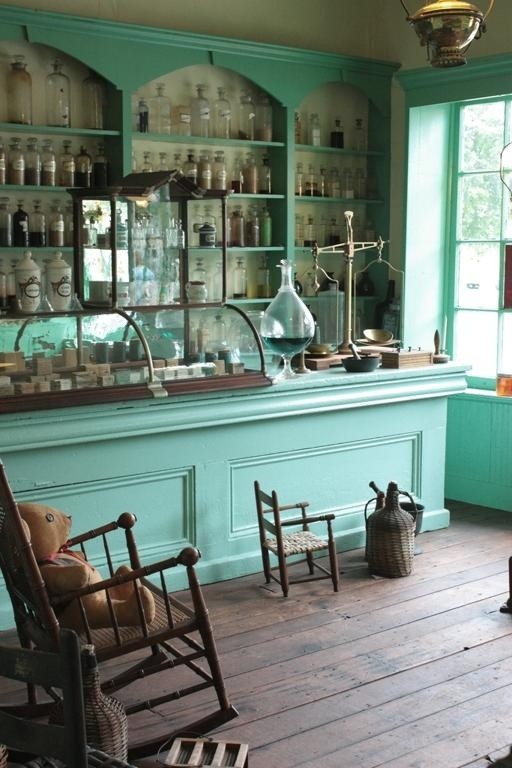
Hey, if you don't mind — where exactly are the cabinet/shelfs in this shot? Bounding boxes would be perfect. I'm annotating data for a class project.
[0,5,401,362]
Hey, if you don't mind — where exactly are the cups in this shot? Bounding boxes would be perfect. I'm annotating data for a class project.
[13,249,73,315]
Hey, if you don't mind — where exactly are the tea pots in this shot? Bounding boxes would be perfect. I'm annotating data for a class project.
[185,282,208,305]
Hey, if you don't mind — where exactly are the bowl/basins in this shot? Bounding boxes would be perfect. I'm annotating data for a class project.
[341,357,380,373]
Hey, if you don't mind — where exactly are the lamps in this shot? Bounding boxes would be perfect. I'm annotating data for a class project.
[400,0,494,68]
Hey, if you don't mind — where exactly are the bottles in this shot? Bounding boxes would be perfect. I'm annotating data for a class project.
[191,204,275,249]
[295,163,370,199]
[261,258,316,384]
[192,256,272,301]
[293,271,303,296]
[291,112,369,150]
[376,278,395,331]
[355,267,373,296]
[132,147,273,196]
[0,256,53,315]
[189,313,238,357]
[8,54,109,131]
[0,136,110,187]
[321,271,339,291]
[1,197,77,246]
[130,84,284,143]
[296,212,379,250]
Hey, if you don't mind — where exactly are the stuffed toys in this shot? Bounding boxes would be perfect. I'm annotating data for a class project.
[13,502,156,628]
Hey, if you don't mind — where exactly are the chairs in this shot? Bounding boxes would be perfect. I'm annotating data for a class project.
[0,459,240,761]
[254,480,338,597]
[0,628,134,768]
